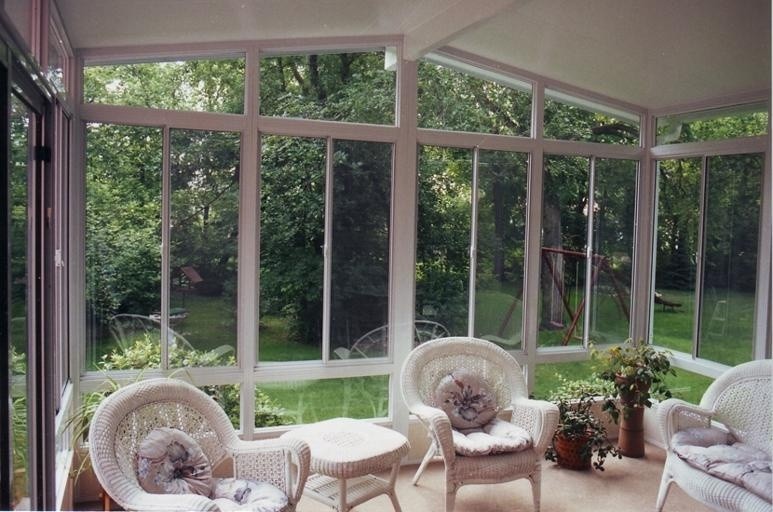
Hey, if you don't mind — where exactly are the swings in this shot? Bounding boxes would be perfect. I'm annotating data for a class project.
[548,251,585,340]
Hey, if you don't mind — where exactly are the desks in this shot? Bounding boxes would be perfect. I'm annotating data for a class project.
[279,417,411,512]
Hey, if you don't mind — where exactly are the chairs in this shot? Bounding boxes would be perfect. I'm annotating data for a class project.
[87,381,311,511]
[652,358,772,512]
[332,317,451,417]
[452,289,522,349]
[107,314,235,368]
[400,334,561,512]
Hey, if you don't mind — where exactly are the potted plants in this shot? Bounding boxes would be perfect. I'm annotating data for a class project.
[540,370,624,472]
[587,336,678,425]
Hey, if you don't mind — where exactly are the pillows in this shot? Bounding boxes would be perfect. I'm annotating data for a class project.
[136,427,213,498]
[433,368,498,431]
